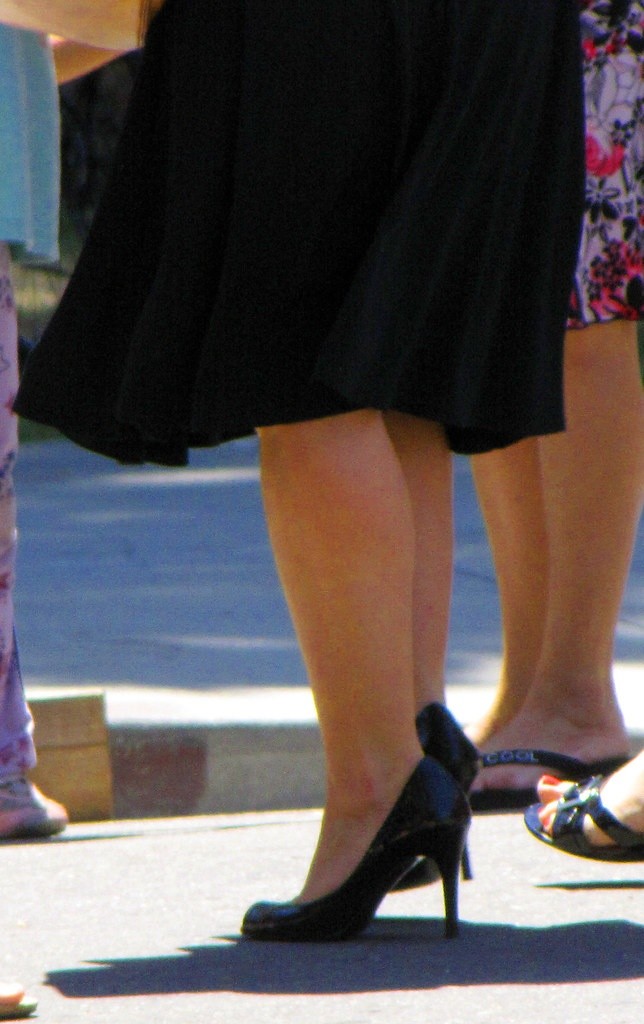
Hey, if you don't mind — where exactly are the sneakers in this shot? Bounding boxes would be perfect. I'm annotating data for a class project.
[0,777,68,835]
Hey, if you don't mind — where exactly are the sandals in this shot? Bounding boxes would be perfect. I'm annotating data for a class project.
[524,774,644,861]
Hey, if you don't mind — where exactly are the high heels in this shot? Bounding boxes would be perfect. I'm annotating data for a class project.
[243,758,475,944]
[396,705,480,891]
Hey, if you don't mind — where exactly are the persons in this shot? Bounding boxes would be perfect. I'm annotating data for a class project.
[0,0,589,942]
[468,0,644,862]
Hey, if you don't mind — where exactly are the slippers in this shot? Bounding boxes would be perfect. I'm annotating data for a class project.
[469,747,632,811]
